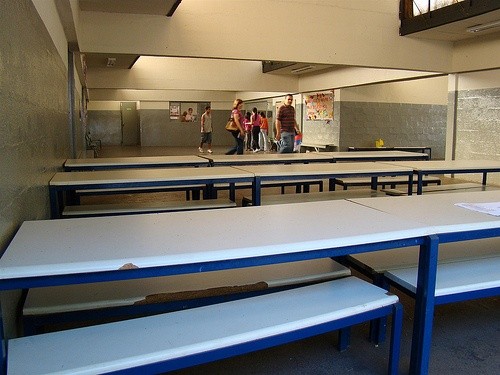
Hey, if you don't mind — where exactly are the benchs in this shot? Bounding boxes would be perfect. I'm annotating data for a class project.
[0,131,500,374]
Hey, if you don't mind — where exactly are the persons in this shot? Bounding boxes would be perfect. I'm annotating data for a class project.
[245,107,261,152]
[258,112,269,151]
[242,112,253,151]
[184,108,194,121]
[224,99,247,155]
[276,93,300,154]
[198,106,213,153]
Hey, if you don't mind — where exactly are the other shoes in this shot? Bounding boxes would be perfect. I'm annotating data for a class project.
[246,148,270,153]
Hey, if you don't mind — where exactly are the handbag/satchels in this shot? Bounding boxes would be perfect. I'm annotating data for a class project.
[225,118,244,132]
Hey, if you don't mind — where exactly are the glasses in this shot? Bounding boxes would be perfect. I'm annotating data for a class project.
[286,99,293,101]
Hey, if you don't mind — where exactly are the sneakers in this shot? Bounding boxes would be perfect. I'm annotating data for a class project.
[198,147,204,153]
[207,148,213,153]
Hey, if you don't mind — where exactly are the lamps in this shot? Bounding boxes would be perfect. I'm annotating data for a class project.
[106,57,116,69]
